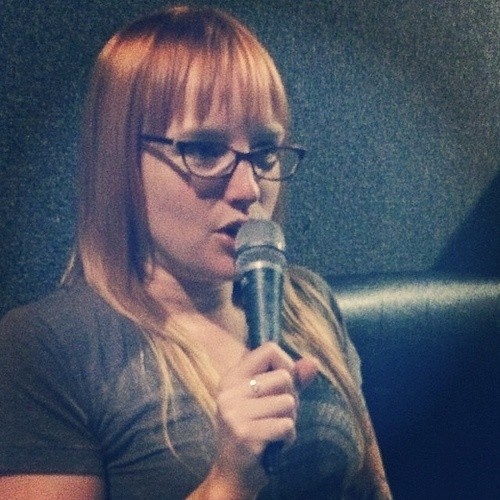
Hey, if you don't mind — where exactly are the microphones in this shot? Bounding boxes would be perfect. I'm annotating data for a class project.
[232,218,290,473]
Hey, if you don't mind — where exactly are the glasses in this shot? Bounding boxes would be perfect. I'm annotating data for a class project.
[141,132,308,179]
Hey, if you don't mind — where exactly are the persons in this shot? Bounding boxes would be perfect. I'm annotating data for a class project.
[0,6,391,500]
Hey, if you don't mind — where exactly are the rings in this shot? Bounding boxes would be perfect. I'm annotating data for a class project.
[250,375,260,398]
